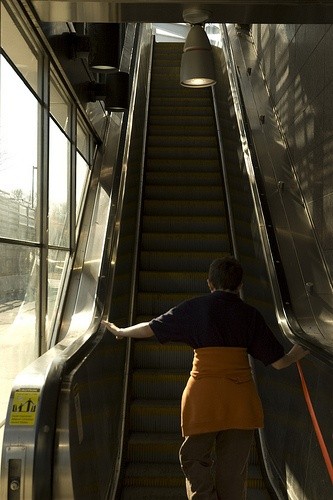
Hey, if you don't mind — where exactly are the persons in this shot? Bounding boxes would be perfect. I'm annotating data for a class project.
[99,254,311,500]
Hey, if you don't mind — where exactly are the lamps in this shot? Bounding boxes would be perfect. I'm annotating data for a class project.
[86,72,131,112]
[177,7,217,89]
[59,31,118,71]
